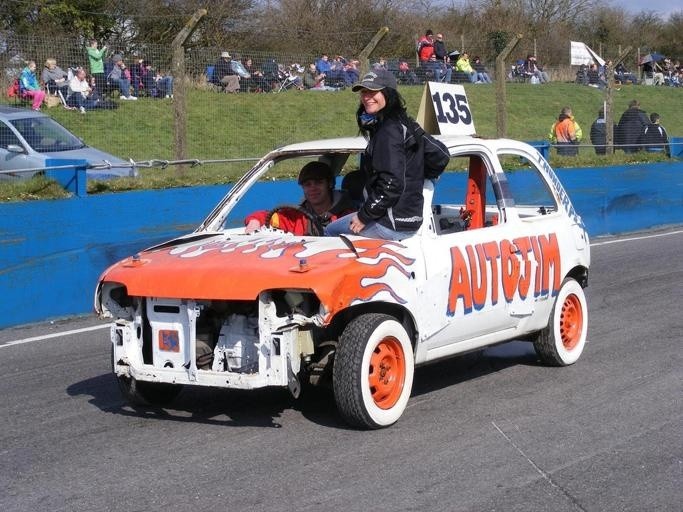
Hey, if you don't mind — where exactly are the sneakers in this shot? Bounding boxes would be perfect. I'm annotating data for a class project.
[120,96,137,100]
[79,105,86,114]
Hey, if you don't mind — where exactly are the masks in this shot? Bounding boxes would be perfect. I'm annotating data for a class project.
[232,53,242,61]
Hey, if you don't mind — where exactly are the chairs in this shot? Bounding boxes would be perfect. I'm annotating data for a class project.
[23,127,42,145]
[341,168,363,199]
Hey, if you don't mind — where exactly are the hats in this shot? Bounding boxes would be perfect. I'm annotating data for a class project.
[298,161,332,184]
[352,67,396,93]
[220,51,231,58]
[425,30,433,35]
[112,54,122,63]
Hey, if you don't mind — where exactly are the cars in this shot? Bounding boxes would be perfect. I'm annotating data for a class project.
[91,132,594,432]
[0,104,139,193]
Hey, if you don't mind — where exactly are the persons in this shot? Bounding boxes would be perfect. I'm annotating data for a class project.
[513,53,609,90]
[614,57,681,87]
[242,161,358,240]
[370,28,493,85]
[15,40,174,114]
[321,66,450,240]
[641,112,669,154]
[213,51,361,93]
[616,98,650,154]
[589,105,618,155]
[547,116,582,147]
[554,106,577,156]
